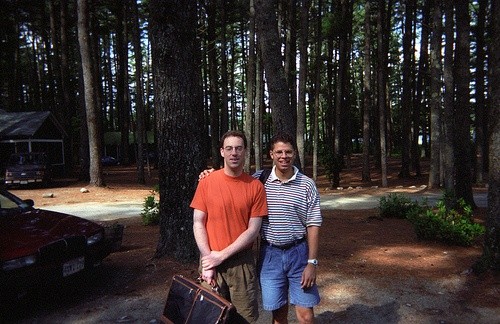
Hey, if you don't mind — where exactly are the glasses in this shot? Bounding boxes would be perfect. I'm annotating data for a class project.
[272,150,295,157]
[223,147,244,152]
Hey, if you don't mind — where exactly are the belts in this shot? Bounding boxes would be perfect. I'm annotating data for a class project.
[262,236,305,250]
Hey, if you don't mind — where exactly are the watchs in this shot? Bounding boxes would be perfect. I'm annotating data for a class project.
[307,259,319,265]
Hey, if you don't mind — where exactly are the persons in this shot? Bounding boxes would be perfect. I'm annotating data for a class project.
[197,134,322,324]
[189,130,269,324]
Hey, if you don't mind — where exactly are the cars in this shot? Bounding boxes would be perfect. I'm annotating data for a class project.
[4,151,52,187]
[0,187,115,302]
[101,155,120,166]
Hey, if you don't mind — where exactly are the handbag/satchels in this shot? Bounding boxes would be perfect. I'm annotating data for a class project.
[160,274,233,324]
